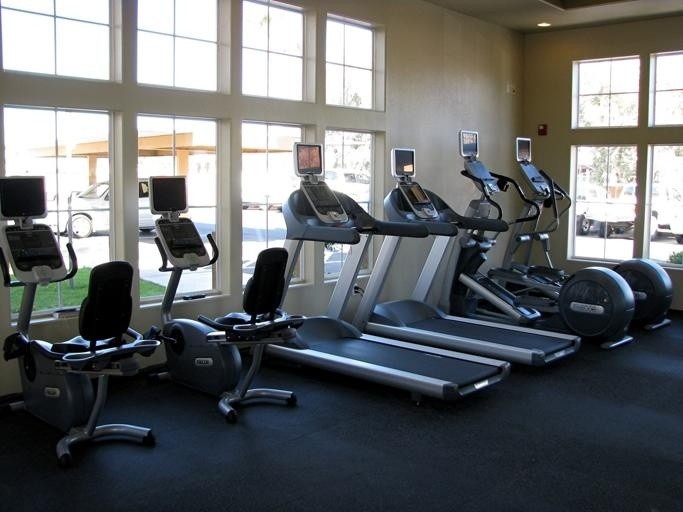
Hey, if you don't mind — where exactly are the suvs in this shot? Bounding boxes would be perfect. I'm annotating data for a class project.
[322,166,372,206]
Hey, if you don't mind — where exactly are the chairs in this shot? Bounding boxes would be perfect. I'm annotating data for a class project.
[212,246,299,424]
[29,259,161,472]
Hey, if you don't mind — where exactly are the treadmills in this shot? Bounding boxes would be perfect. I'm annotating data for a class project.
[353,148,581,371]
[266,143,513,400]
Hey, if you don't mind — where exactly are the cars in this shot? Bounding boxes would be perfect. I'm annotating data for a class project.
[31,176,163,239]
[572,159,681,251]
